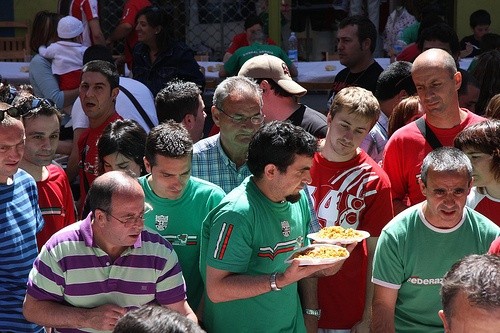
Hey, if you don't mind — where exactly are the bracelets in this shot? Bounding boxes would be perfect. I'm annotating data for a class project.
[270,271,282,291]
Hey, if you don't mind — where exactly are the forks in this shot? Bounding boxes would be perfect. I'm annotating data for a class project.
[465,42,479,50]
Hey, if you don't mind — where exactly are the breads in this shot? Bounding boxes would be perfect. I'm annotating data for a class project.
[208,64,223,71]
[325,65,336,71]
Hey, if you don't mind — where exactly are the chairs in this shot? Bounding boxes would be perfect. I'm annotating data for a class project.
[325,51,340,101]
[0,19,33,62]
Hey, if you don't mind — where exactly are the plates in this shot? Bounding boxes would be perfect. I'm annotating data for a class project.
[461,57,473,62]
[284,243,350,265]
[307,229,371,245]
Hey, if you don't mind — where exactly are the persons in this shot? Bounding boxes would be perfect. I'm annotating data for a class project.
[298,87,396,333]
[22,170,198,333]
[370,147,500,333]
[189,76,267,195]
[0,102,52,333]
[82,118,148,218]
[114,305,203,333]
[454,121,500,223]
[73,60,127,219]
[437,252,499,333]
[0,1,500,192]
[129,121,228,321]
[13,95,76,250]
[197,121,360,333]
[379,49,494,215]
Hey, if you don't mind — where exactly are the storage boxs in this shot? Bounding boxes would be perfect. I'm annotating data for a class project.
[297,37,313,53]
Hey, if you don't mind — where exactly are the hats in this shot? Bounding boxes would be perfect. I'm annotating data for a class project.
[236,53,307,97]
[57,16,84,39]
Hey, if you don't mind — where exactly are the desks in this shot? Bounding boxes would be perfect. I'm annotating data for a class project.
[0,56,474,92]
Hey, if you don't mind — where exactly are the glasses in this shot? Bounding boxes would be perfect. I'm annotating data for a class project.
[218,108,264,124]
[99,208,151,228]
[0,107,20,122]
[21,98,56,114]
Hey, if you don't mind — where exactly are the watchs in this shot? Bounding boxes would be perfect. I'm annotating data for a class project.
[304,308,321,321]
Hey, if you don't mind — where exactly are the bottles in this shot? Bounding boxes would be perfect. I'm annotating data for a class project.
[287,32,298,68]
[394,27,404,53]
